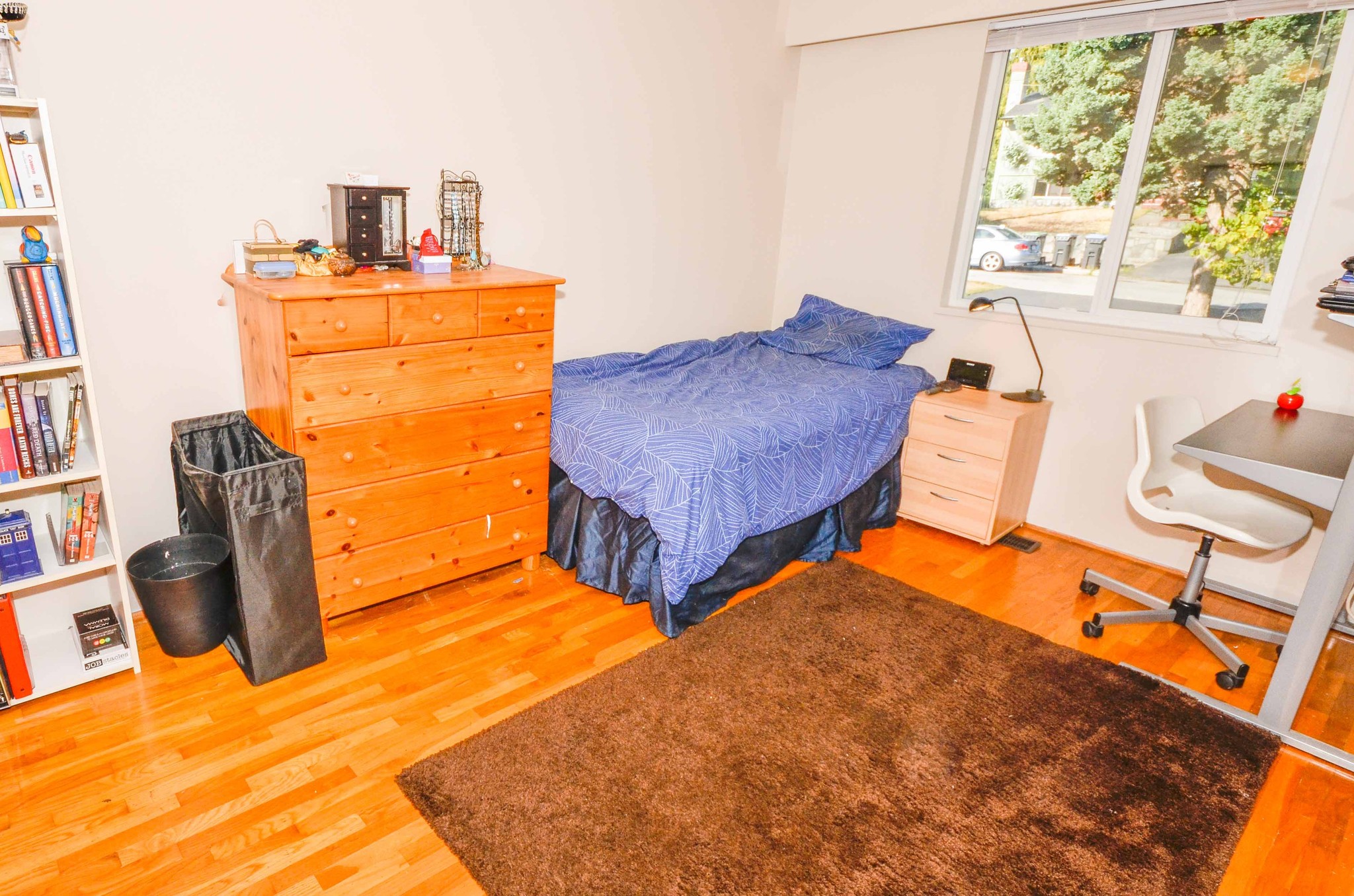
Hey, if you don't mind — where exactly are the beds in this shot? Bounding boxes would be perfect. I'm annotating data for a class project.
[540,330,937,639]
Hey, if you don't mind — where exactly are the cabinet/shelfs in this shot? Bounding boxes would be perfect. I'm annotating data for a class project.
[0,96,143,710]
[326,183,412,272]
[896,386,1053,546]
[219,264,567,635]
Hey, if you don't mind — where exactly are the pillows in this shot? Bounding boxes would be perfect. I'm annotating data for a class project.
[757,293,935,370]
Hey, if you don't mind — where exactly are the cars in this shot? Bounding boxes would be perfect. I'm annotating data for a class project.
[969,224,1043,272]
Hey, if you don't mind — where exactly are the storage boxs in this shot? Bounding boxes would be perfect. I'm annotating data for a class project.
[252,261,297,280]
[72,604,123,656]
[69,626,131,673]
[410,253,453,274]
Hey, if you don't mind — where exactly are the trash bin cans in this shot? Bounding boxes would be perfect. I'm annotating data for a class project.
[124,532,234,658]
[1080,234,1107,270]
[1052,232,1078,269]
[1024,233,1047,266]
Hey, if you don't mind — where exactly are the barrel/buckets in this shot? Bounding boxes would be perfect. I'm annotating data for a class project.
[124,532,232,658]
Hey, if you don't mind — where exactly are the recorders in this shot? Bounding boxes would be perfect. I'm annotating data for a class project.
[947,358,995,390]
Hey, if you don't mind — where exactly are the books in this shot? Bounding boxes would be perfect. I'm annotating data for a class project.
[1,127,102,586]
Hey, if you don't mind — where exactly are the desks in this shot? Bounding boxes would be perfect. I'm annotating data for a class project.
[1116,310,1354,774]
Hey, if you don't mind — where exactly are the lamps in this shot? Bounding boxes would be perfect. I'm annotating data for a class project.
[968,296,1047,403]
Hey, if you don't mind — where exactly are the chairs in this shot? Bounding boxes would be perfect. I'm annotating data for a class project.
[1078,395,1316,690]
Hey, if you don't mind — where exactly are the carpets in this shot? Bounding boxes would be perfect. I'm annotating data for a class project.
[394,555,1282,896]
